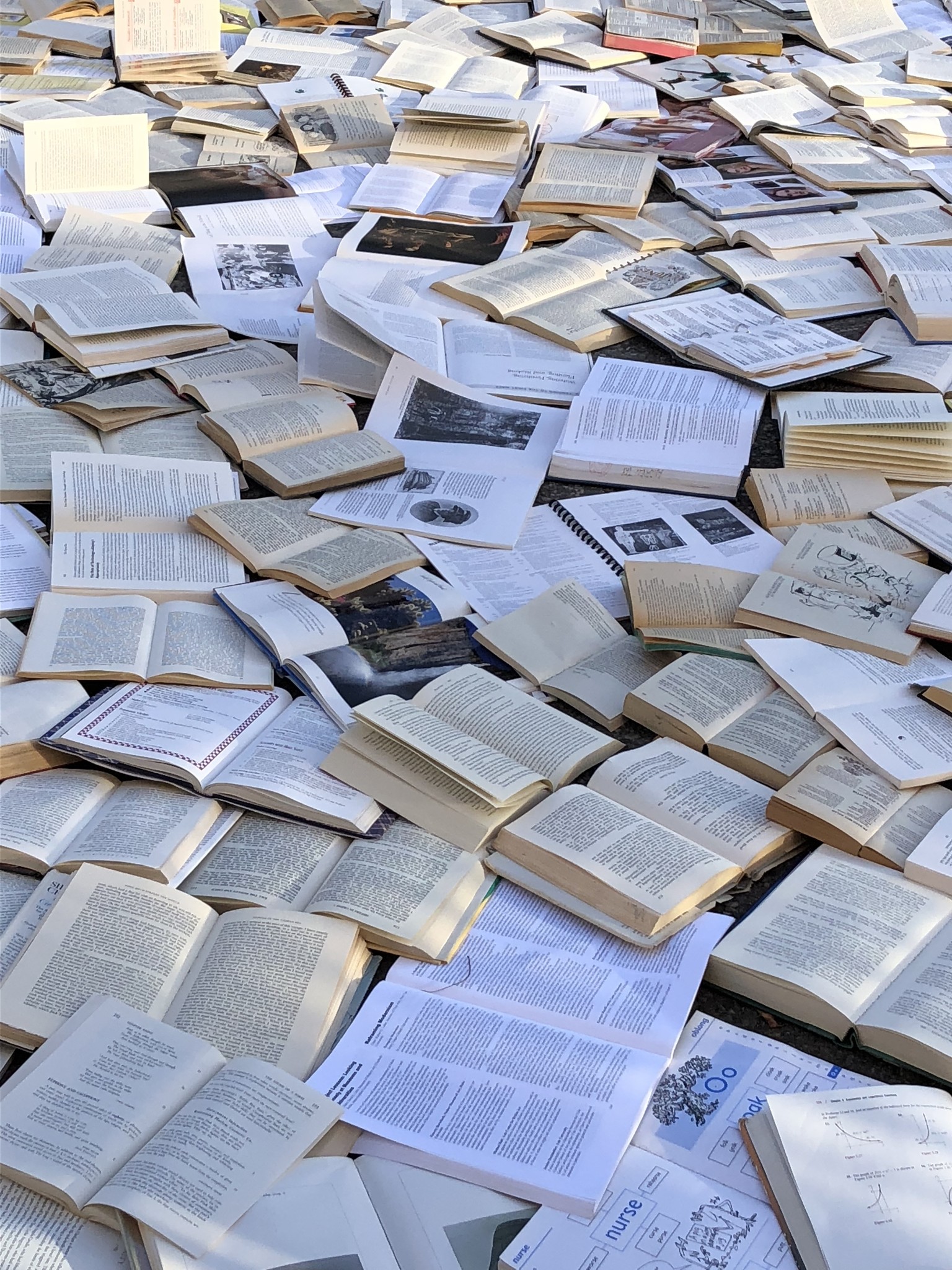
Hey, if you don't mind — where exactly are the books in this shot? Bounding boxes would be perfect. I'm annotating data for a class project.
[0,0,952,1269]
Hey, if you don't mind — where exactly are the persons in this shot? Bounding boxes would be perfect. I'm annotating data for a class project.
[771,187,822,200]
[715,160,789,173]
